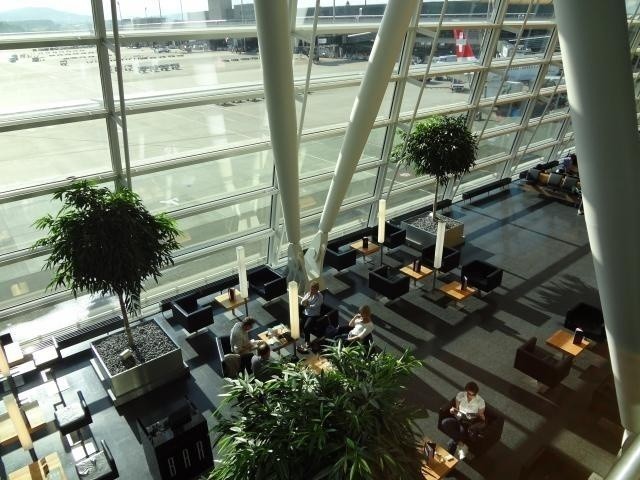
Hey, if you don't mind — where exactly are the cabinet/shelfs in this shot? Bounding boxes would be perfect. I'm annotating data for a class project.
[135,402,216,478]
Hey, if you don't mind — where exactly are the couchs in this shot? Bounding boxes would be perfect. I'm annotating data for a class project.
[215,304,372,383]
[170,292,214,338]
[248,265,287,307]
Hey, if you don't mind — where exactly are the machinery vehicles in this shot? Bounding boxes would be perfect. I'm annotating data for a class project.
[107,48,189,73]
[8,45,99,68]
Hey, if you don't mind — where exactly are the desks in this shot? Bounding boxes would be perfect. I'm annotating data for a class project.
[544,328,590,360]
[214,289,249,321]
[418,435,460,480]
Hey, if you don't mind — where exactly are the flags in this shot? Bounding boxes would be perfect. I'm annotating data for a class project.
[453,28,478,69]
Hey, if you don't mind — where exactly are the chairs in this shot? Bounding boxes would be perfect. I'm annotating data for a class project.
[563,303,606,344]
[323,220,504,310]
[53,390,100,462]
[31,334,72,413]
[437,393,506,461]
[75,440,121,480]
[519,154,583,215]
[514,336,573,388]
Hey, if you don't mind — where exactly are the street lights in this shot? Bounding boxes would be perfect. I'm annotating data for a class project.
[117,1,123,25]
[144,8,148,23]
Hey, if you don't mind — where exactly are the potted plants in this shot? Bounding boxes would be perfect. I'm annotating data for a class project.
[390,109,482,250]
[26,175,192,409]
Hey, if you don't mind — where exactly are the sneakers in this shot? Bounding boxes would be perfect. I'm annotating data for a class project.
[459,444,469,460]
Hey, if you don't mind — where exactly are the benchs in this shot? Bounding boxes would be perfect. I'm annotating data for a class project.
[388,199,452,225]
[461,176,512,205]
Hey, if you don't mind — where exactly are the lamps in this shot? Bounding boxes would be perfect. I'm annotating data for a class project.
[378,199,385,266]
[236,245,254,327]
[288,280,299,363]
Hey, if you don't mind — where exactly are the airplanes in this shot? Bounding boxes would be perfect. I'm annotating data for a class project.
[452,26,566,88]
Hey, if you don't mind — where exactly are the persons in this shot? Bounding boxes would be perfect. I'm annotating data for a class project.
[315,303,375,348]
[300,281,325,351]
[438,382,487,455]
[249,343,282,379]
[227,315,256,364]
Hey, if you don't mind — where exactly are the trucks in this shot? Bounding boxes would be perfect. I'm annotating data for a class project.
[435,54,457,64]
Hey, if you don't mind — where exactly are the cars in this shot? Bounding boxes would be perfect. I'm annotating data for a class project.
[416,76,433,82]
[435,75,450,82]
[450,77,465,92]
[413,56,423,64]
